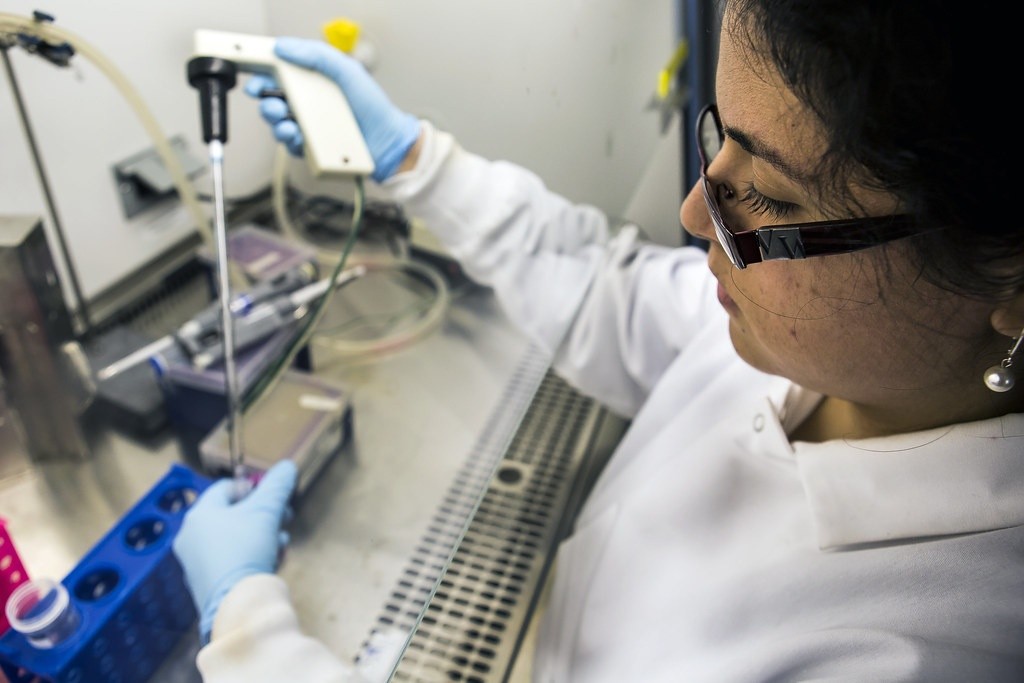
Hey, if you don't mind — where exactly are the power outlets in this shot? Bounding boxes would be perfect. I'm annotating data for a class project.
[116,136,206,219]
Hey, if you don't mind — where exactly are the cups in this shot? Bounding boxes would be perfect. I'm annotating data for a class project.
[5,574,82,649]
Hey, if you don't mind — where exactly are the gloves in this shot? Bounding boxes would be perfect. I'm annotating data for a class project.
[172,459,300,646]
[243,34,418,180]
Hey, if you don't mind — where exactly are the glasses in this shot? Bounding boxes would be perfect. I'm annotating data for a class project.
[694,100,924,272]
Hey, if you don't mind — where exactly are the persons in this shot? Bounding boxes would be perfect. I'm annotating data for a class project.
[171,0,1024,683]
